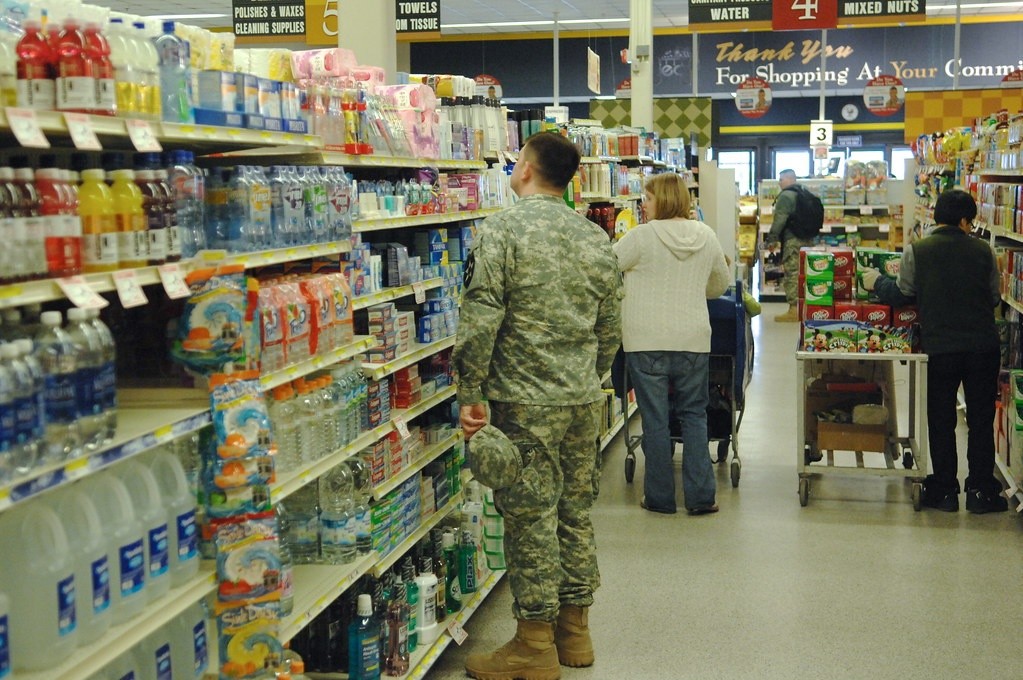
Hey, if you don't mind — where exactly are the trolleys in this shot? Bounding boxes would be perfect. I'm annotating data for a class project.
[795,320,929,511]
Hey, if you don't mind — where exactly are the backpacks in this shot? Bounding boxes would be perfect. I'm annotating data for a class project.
[780,186,825,240]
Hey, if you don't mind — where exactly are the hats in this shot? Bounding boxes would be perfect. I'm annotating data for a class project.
[466,424,523,490]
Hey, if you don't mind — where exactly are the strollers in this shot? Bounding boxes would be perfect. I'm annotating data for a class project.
[609,280,762,488]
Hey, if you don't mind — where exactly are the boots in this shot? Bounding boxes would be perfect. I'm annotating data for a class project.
[465,618,561,680]
[553,606,595,666]
[774,306,798,322]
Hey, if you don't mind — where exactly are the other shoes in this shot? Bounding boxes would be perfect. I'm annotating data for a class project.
[640,495,677,513]
[686,501,719,514]
[966,491,1009,513]
[911,489,959,512]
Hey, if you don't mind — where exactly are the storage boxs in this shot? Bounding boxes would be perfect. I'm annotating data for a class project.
[758,181,888,205]
[255,219,488,566]
[799,246,920,354]
[618,135,642,156]
[419,168,481,211]
[807,380,886,451]
[198,70,298,119]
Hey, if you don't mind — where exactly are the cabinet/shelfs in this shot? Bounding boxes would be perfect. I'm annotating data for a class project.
[795,350,929,511]
[697,146,759,288]
[0,108,701,680]
[910,162,1023,509]
[754,182,903,303]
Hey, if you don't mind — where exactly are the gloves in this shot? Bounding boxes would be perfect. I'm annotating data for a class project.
[862,266,882,290]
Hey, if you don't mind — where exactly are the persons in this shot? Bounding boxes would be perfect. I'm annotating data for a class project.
[456,132,625,680]
[614,172,730,514]
[886,86,903,108]
[487,86,497,99]
[767,169,814,323]
[863,189,1008,513]
[755,89,769,111]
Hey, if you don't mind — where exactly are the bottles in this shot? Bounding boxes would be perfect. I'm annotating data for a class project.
[0,0,461,680]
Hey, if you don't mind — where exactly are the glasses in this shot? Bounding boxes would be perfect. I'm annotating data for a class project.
[966,220,977,231]
